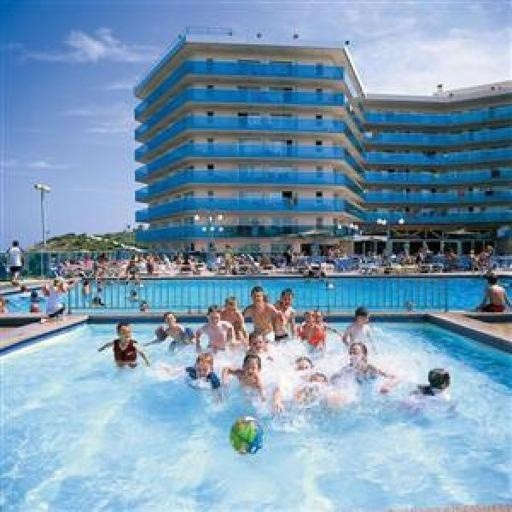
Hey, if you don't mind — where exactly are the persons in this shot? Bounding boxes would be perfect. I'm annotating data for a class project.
[187,246,496,278]
[343,305,378,357]
[409,368,452,397]
[183,353,221,408]
[220,297,249,348]
[476,273,512,313]
[311,308,344,350]
[196,304,235,353]
[98,322,150,370]
[245,331,266,356]
[7,241,24,286]
[295,373,329,408]
[144,312,194,355]
[330,343,403,393]
[297,310,324,355]
[271,357,313,415]
[222,354,267,408]
[272,288,298,342]
[241,287,283,342]
[28,251,185,315]
[1,297,9,315]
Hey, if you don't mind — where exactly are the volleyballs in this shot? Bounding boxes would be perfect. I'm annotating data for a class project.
[230,417,264,454]
